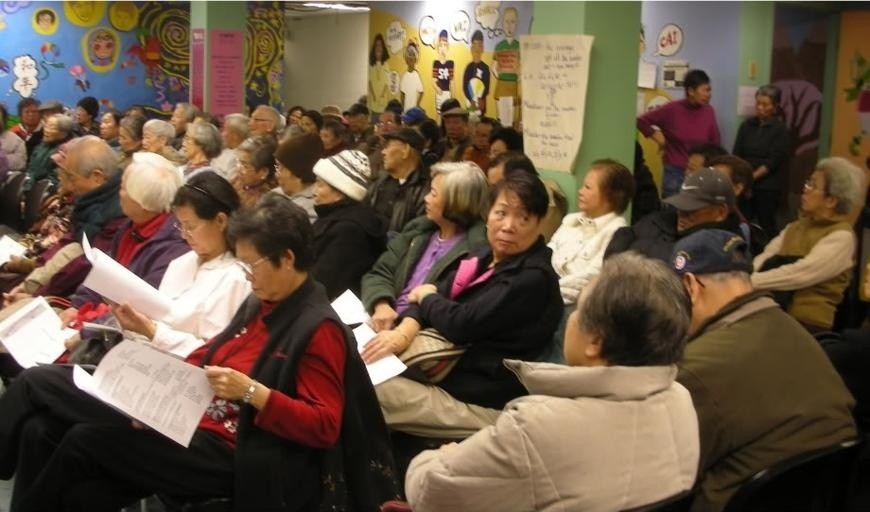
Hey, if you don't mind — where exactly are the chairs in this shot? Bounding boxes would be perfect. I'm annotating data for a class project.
[723,436,869,510]
[620,488,694,512]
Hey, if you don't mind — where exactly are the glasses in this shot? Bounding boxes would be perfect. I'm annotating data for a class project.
[238,257,270,276]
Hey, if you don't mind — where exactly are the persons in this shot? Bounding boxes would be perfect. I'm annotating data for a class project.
[729,85,785,223]
[636,69,722,201]
[369,6,524,129]
[1,96,869,512]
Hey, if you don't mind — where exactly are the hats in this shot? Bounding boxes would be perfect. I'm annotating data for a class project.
[35,101,62,111]
[76,97,98,117]
[441,107,469,117]
[669,228,753,274]
[660,168,733,211]
[399,108,425,122]
[320,105,343,119]
[272,128,425,202]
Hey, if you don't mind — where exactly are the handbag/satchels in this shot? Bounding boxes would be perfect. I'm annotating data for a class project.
[396,326,468,386]
